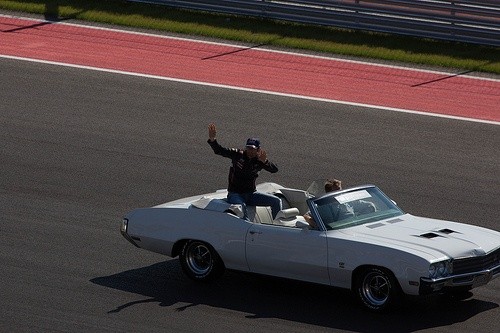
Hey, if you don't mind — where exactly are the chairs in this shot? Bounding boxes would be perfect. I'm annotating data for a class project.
[205,189,306,227]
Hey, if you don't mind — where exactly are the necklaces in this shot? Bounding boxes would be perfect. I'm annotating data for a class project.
[329,204,340,222]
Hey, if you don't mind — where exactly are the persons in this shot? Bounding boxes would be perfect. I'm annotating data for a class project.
[303,179,376,229]
[207,123,282,222]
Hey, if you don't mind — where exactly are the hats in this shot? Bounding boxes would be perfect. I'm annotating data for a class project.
[246,138,260,149]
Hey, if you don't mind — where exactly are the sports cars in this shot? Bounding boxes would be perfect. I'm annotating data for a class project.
[120,184,500,315]
